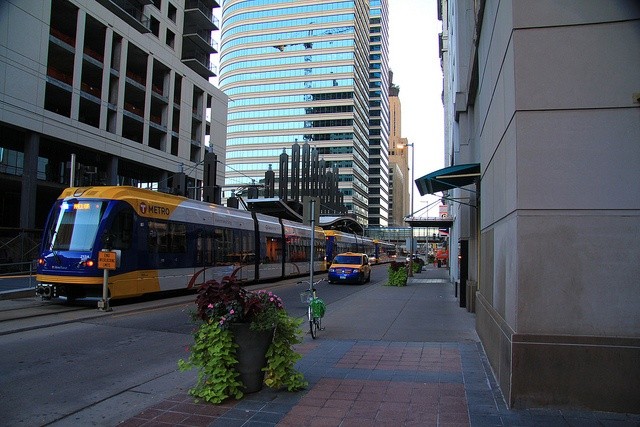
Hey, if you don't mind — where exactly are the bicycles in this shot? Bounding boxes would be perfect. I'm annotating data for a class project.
[296,278,330,339]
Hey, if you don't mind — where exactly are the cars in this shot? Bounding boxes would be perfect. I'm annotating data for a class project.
[406,253,418,263]
[329,251,370,284]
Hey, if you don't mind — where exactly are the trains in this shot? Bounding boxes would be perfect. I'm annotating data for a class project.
[36,187,400,305]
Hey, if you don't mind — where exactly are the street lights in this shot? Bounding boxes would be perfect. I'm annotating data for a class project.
[421,200,428,264]
[397,142,414,275]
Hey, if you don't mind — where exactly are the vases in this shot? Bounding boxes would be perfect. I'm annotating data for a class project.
[230,323,274,393]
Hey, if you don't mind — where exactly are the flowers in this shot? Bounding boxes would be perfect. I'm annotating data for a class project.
[179,267,308,405]
